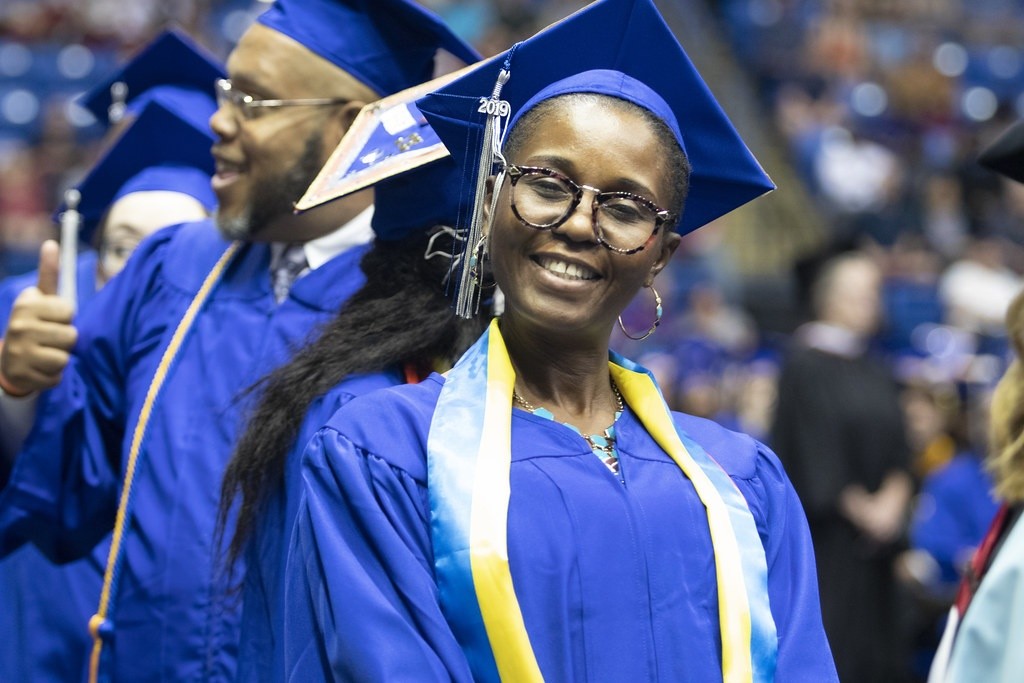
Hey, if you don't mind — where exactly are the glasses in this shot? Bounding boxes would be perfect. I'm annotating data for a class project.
[210,72,354,128]
[492,160,679,255]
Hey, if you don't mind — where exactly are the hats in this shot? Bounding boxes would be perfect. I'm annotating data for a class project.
[295,1,775,271]
[52,98,226,245]
[71,25,233,142]
[254,0,484,97]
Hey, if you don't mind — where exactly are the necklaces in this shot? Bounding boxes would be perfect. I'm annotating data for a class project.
[511,382,631,483]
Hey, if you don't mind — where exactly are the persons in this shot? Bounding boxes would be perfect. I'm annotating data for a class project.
[1,0,1024,561]
[0,2,482,683]
[925,271,1024,683]
[287,0,843,682]
[764,238,914,682]
[205,57,503,678]
